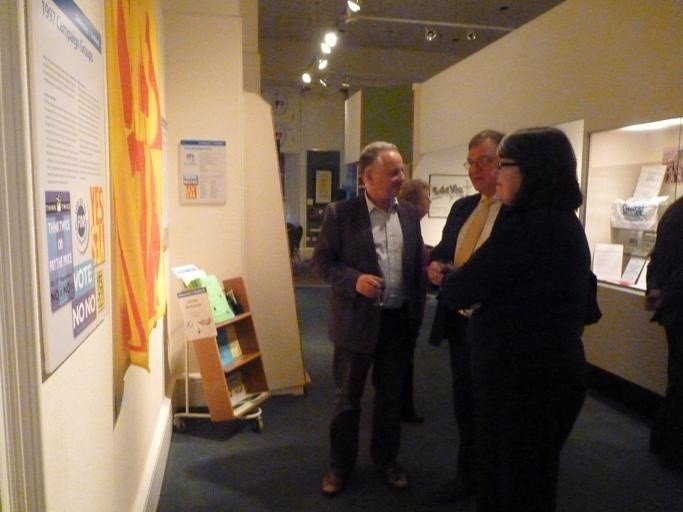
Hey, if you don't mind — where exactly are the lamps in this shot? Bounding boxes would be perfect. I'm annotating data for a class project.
[319,74,328,87]
[341,74,351,88]
[424,24,437,42]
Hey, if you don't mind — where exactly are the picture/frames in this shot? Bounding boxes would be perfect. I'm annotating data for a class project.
[427,172,480,219]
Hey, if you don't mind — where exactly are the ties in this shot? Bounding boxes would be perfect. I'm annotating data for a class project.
[455,195,498,269]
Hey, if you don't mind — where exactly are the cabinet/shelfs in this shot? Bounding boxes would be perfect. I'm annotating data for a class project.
[191,277,272,423]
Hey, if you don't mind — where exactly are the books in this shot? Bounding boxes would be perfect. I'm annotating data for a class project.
[172,264,247,406]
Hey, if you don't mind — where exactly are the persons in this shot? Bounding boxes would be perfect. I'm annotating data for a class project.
[426,130,512,504]
[313,141,430,493]
[644,194,683,469]
[398,178,434,424]
[437,128,603,512]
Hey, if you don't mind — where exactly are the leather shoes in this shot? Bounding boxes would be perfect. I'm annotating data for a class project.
[322,472,343,493]
[402,410,424,423]
[385,468,407,487]
[432,479,479,502]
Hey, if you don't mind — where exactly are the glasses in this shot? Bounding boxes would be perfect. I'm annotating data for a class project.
[498,161,518,169]
[464,159,497,170]
